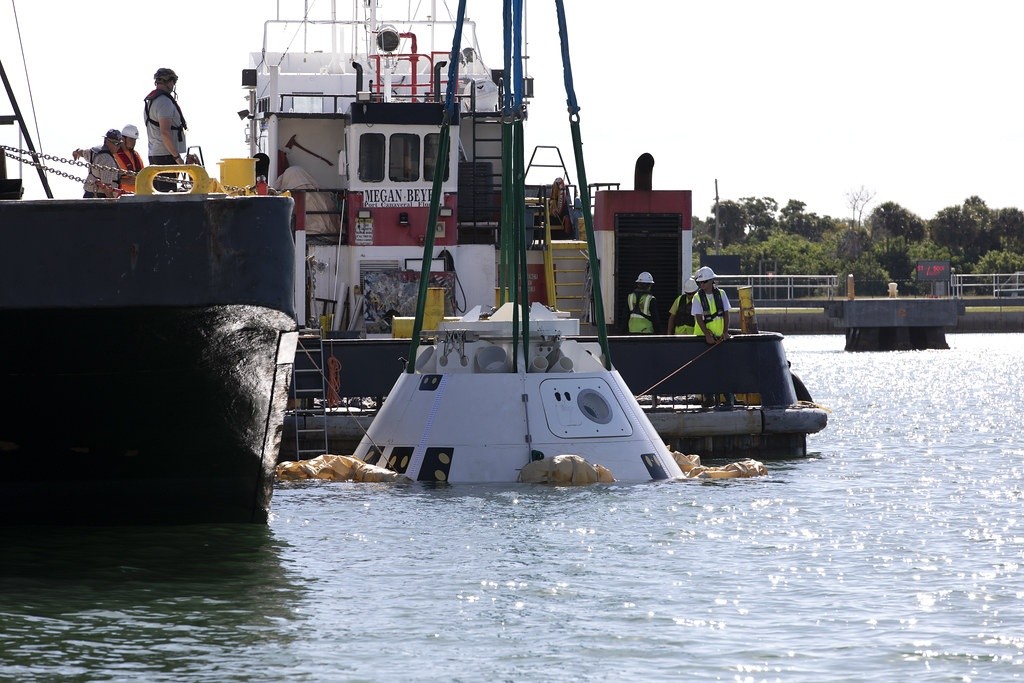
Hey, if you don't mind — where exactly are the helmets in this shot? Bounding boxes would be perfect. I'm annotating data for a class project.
[154,69,178,85]
[636,272,655,283]
[695,269,700,283]
[121,125,140,139]
[684,279,699,293]
[696,267,716,281]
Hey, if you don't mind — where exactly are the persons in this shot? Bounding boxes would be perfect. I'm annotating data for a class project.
[627,272,659,336]
[72,129,124,198]
[111,125,144,195]
[667,278,699,335]
[144,68,187,192]
[691,267,731,344]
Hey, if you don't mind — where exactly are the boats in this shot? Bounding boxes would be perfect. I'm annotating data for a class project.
[232,0,831,493]
[0,53,302,530]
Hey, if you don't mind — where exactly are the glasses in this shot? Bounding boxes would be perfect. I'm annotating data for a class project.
[700,279,713,284]
[696,281,700,284]
[107,139,122,145]
[685,292,697,295]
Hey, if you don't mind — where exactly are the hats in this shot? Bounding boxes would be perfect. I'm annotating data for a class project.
[107,129,121,140]
